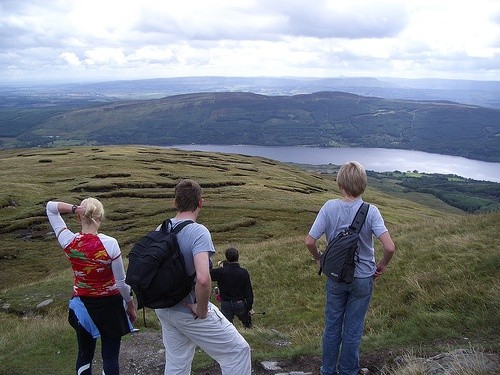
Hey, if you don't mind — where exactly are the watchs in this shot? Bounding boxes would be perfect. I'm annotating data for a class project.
[71,204,78,214]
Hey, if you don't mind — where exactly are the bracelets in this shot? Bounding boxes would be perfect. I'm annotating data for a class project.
[311,250,323,260]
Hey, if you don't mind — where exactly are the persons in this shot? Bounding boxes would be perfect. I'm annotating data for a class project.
[44,197,139,375]
[154,178,254,375]
[303,160,396,375]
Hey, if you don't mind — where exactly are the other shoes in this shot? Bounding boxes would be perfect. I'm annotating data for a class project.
[356,367,370,375]
[319,366,324,375]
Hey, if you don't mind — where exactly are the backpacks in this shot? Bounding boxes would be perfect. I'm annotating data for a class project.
[123,219,197,328]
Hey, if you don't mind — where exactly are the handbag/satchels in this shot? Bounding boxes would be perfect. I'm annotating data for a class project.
[316,201,370,285]
